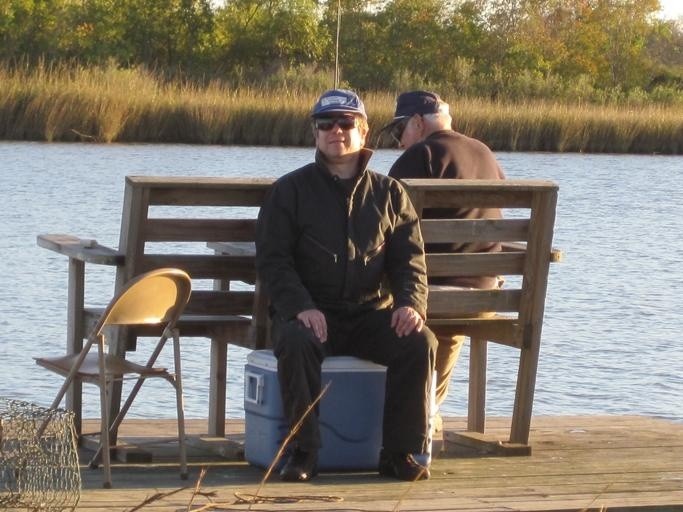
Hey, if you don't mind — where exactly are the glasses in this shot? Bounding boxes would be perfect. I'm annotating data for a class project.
[316,119,355,130]
[390,118,408,141]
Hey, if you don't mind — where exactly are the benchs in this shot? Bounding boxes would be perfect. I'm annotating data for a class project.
[35,174,561,462]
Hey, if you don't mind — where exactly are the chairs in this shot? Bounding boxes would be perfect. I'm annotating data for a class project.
[32,269,192,490]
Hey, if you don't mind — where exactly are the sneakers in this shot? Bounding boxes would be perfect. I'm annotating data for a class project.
[379,449,428,481]
[280,451,317,481]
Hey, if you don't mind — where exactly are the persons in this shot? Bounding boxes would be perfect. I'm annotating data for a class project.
[253,90,439,483]
[378,90,508,437]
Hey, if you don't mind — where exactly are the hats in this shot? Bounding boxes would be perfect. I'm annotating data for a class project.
[379,91,450,132]
[311,89,367,117]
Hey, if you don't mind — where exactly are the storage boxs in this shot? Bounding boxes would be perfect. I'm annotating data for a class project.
[243,351,388,468]
